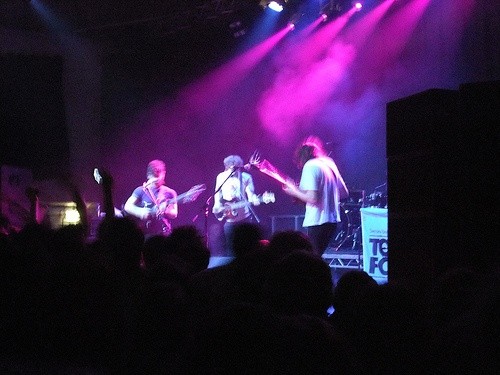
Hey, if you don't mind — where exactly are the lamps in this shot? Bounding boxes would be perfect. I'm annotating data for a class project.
[318,2,335,22]
[287,12,302,31]
[264,0,288,13]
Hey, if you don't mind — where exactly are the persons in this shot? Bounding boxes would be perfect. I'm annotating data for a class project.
[124,160,178,240]
[212,155,261,251]
[0,169,500,375]
[281,137,349,257]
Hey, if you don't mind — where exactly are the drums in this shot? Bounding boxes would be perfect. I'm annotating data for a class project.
[329,202,353,247]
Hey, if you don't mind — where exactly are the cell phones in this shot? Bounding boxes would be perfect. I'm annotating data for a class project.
[93,168,102,184]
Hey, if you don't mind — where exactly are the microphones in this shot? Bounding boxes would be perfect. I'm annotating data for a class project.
[234,163,252,171]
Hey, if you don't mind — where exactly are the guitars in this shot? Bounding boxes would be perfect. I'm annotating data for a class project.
[215,191,275,221]
[249,149,301,193]
[134,184,208,229]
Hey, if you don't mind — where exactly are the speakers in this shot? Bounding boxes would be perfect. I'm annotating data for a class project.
[385,79,500,287]
